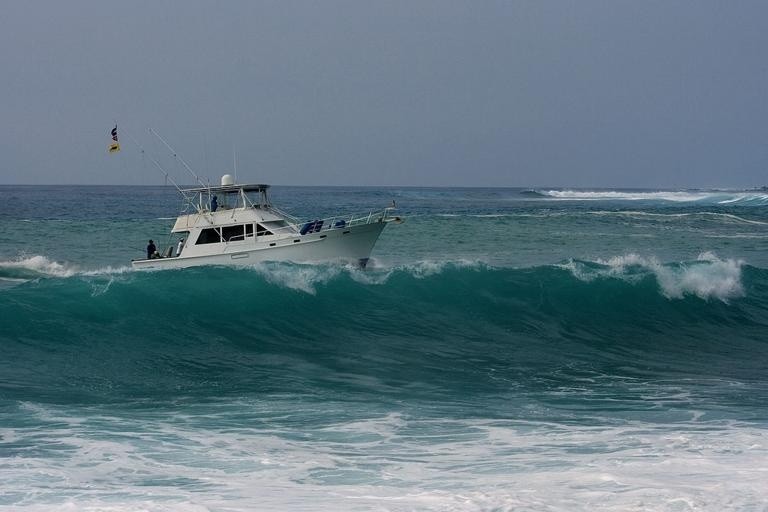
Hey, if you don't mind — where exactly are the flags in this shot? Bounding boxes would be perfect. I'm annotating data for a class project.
[111,127,118,141]
[107,143,121,153]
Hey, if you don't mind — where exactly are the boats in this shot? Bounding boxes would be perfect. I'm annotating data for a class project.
[131,168,401,276]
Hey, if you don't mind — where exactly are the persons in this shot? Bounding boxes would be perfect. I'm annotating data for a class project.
[211,196,218,212]
[147,240,156,259]
[176,237,184,257]
